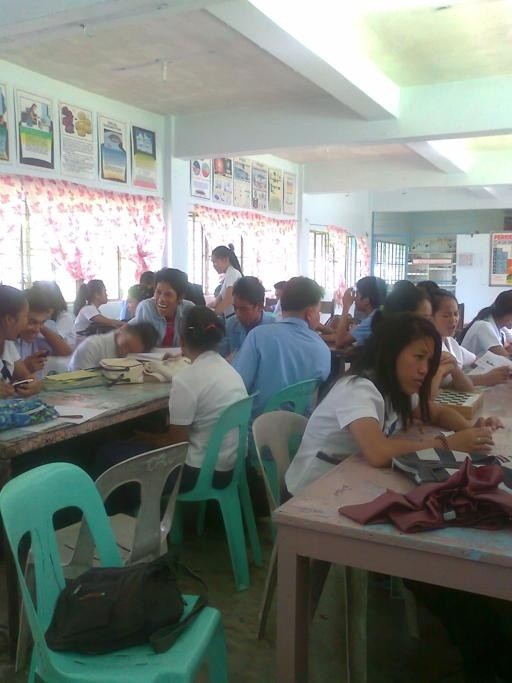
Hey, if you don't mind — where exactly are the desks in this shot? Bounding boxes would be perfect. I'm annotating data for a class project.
[269,380,512,682]
[325,341,355,380]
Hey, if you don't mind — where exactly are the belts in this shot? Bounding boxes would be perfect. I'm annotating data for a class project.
[226,311,235,317]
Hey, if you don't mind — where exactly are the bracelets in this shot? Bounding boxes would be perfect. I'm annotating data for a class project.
[436,433,447,454]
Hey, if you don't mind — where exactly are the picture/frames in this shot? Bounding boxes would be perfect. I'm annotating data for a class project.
[488,230,512,288]
[189,154,296,217]
[0,80,161,193]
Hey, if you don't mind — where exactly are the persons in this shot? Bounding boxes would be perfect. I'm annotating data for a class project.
[277,314,512,682]
[1,265,196,429]
[219,276,388,450]
[86,304,254,516]
[205,245,245,322]
[370,277,512,401]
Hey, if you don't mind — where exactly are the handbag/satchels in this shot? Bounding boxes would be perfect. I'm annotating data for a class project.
[390,449,504,483]
[99,358,144,384]
[0,396,60,432]
[44,554,210,656]
[144,356,191,382]
[325,313,355,333]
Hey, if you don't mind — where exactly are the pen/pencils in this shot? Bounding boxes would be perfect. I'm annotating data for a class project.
[57,414,84,420]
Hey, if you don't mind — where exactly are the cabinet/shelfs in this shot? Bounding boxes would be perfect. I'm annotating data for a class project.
[406,250,456,287]
